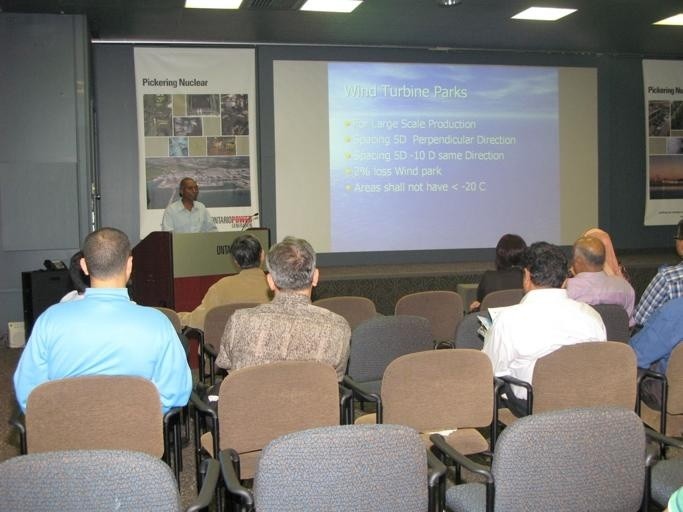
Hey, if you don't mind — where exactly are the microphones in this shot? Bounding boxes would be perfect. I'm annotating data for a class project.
[240,213,258,230]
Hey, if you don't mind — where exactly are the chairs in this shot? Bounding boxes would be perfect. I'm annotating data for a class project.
[0,289,682,512]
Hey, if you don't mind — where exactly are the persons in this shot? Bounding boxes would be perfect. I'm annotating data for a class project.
[161,178,218,233]
[177,235,275,355]
[468,234,528,315]
[565,219,683,412]
[13,228,193,419]
[214,235,351,374]
[479,241,607,418]
